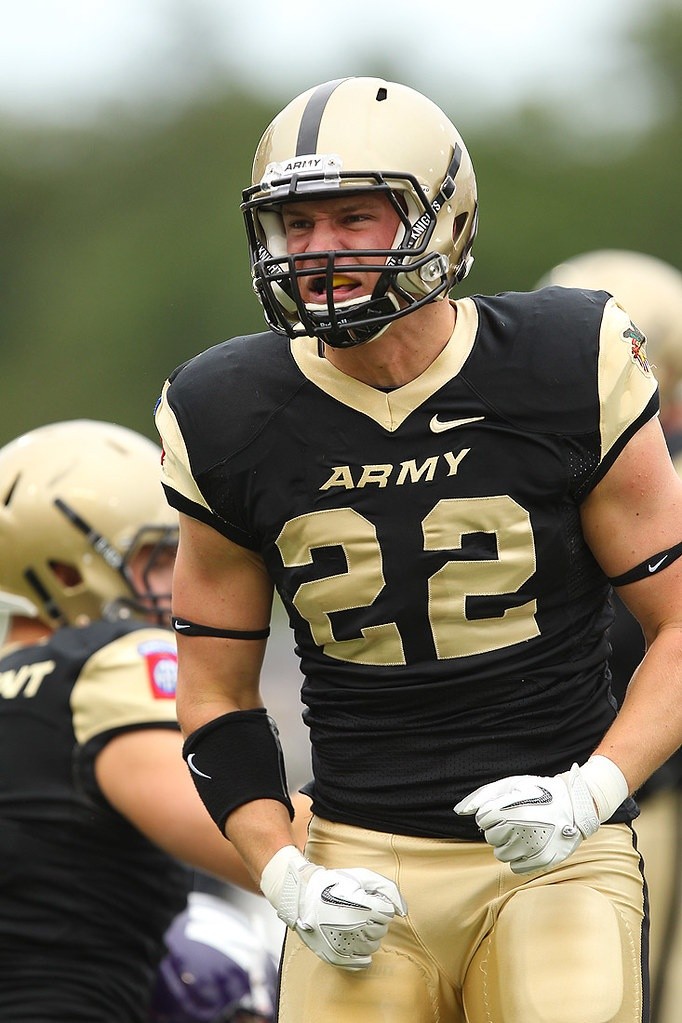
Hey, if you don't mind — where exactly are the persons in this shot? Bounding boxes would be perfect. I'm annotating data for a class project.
[153,77,682,1023]
[537,249,682,1023]
[0,419,314,1023]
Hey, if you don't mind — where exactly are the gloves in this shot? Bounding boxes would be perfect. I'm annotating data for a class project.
[454,755,627,876]
[256,846,405,972]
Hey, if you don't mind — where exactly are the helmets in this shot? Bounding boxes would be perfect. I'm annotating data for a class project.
[548,251,682,462]
[1,420,181,630]
[240,76,478,350]
